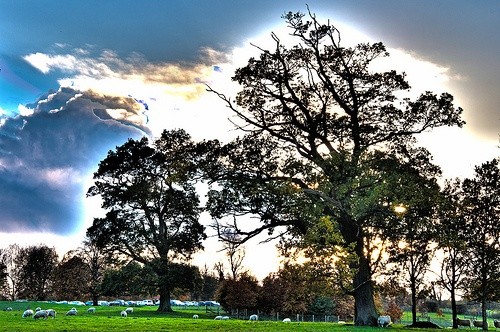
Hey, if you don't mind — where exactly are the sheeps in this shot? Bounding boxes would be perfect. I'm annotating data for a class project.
[282,318,291,323]
[249,314,258,321]
[213,315,230,321]
[455,317,471,330]
[193,314,198,319]
[438,321,450,330]
[86,308,95,313]
[21,306,57,320]
[377,315,393,328]
[7,307,13,311]
[120,307,133,317]
[493,318,500,329]
[65,307,78,316]
[472,319,488,329]
